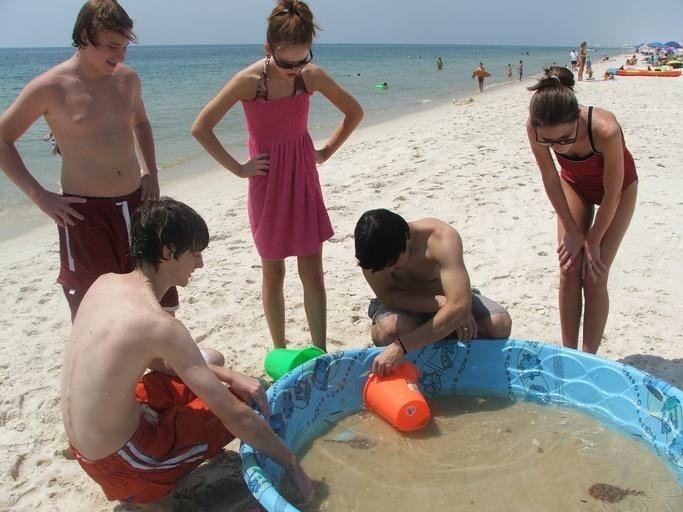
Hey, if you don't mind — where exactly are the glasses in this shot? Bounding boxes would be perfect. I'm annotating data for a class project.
[533,116,580,146]
[268,40,316,68]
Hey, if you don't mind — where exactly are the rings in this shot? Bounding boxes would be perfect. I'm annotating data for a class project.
[464,328,468,332]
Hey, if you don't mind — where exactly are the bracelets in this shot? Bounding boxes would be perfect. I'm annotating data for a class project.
[396,337,408,354]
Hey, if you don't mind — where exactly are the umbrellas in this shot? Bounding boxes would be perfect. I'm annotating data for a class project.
[634,42,683,48]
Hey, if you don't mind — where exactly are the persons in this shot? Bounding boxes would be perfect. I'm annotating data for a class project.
[0,0,180,325]
[190,0,365,354]
[40,131,61,156]
[344,74,360,76]
[383,83,387,86]
[353,208,512,377]
[60,195,316,512]
[526,66,639,356]
[408,41,675,92]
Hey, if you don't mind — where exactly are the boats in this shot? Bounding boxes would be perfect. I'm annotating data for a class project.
[665,59,683,68]
[614,65,680,77]
[584,48,597,52]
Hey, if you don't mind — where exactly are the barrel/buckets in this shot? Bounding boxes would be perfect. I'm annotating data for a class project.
[261,345,327,384]
[362,363,432,433]
